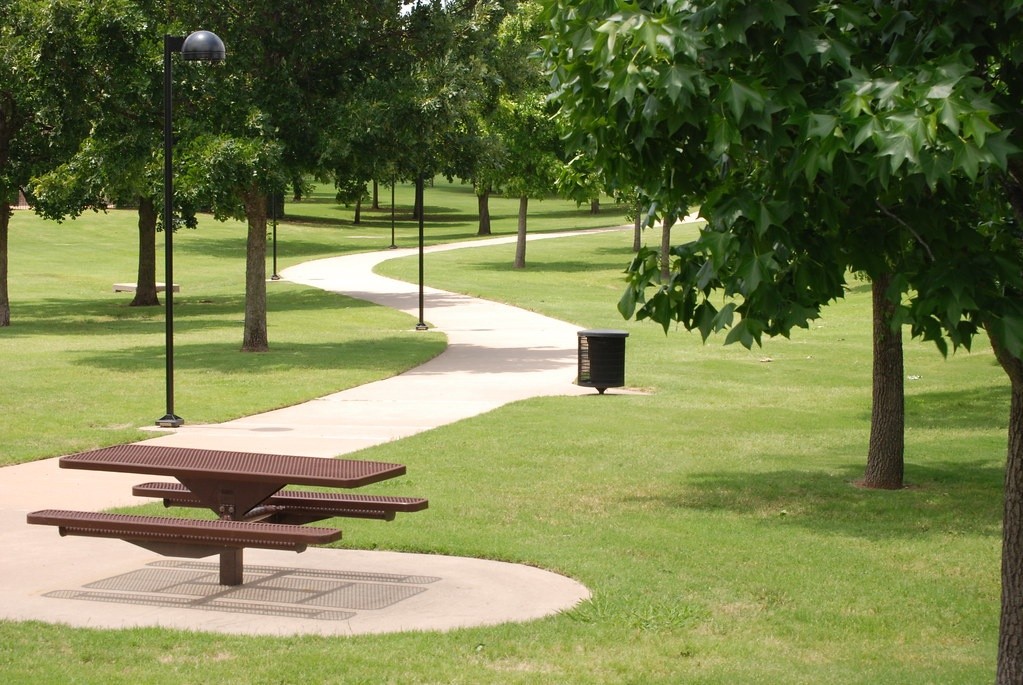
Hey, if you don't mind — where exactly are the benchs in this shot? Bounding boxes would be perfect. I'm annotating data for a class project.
[27,509,342,553]
[132,482,429,522]
[113,282,181,292]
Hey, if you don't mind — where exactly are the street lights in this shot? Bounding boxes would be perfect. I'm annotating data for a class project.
[156,31,225,427]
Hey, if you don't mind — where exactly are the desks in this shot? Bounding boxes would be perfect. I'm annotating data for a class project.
[59,444,406,585]
